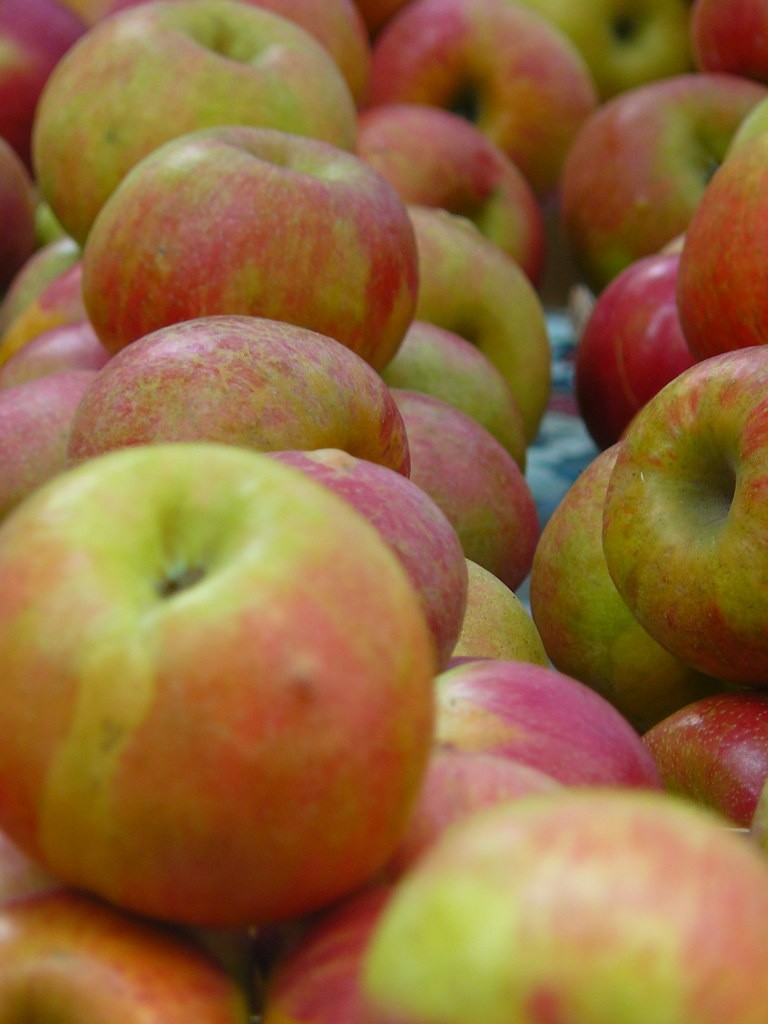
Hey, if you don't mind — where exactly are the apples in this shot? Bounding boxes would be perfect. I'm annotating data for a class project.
[0,0,768,1024]
